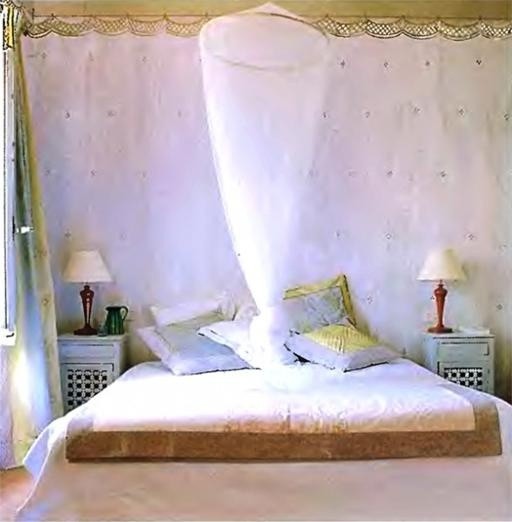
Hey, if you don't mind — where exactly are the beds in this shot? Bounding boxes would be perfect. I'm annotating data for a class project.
[15,271,512,520]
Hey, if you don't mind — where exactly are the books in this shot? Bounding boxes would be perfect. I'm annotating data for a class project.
[457,325,489,335]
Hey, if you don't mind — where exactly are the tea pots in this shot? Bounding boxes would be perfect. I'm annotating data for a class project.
[101,305,128,335]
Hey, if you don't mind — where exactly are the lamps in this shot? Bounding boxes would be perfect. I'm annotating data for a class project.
[62,250,114,335]
[417,248,468,333]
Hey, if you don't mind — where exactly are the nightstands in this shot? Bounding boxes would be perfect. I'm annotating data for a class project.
[56,332,126,416]
[424,327,495,396]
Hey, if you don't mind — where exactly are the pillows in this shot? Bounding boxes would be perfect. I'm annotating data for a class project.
[136,272,407,377]
